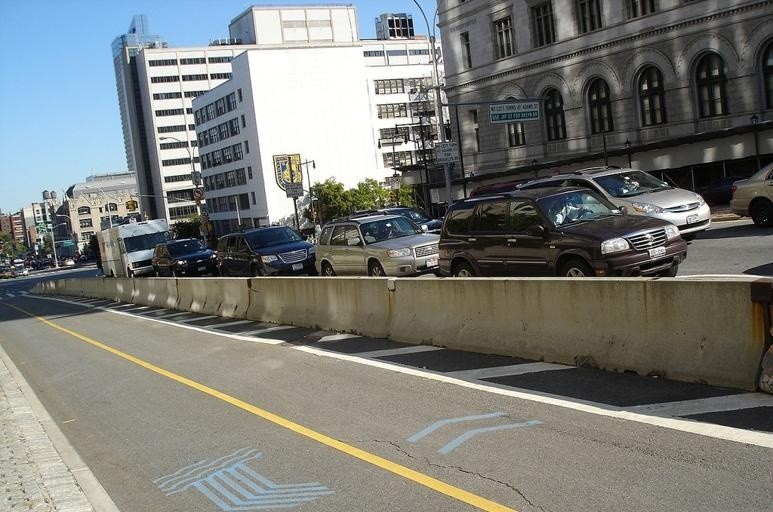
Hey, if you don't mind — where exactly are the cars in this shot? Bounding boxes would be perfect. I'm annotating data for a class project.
[726,163,773,230]
[0,253,85,278]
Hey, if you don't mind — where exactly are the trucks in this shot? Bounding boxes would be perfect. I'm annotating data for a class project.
[95,218,172,280]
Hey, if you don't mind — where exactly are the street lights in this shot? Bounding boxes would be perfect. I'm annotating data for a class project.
[393,116,452,218]
[749,113,760,173]
[376,131,409,178]
[623,137,632,168]
[531,158,539,176]
[49,222,68,268]
[56,180,135,252]
[158,130,217,250]
[297,157,322,243]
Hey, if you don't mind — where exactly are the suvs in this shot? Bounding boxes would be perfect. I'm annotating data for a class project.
[346,207,443,231]
[151,238,218,277]
[515,166,712,242]
[218,226,316,276]
[469,175,557,199]
[431,186,688,280]
[316,214,443,277]
